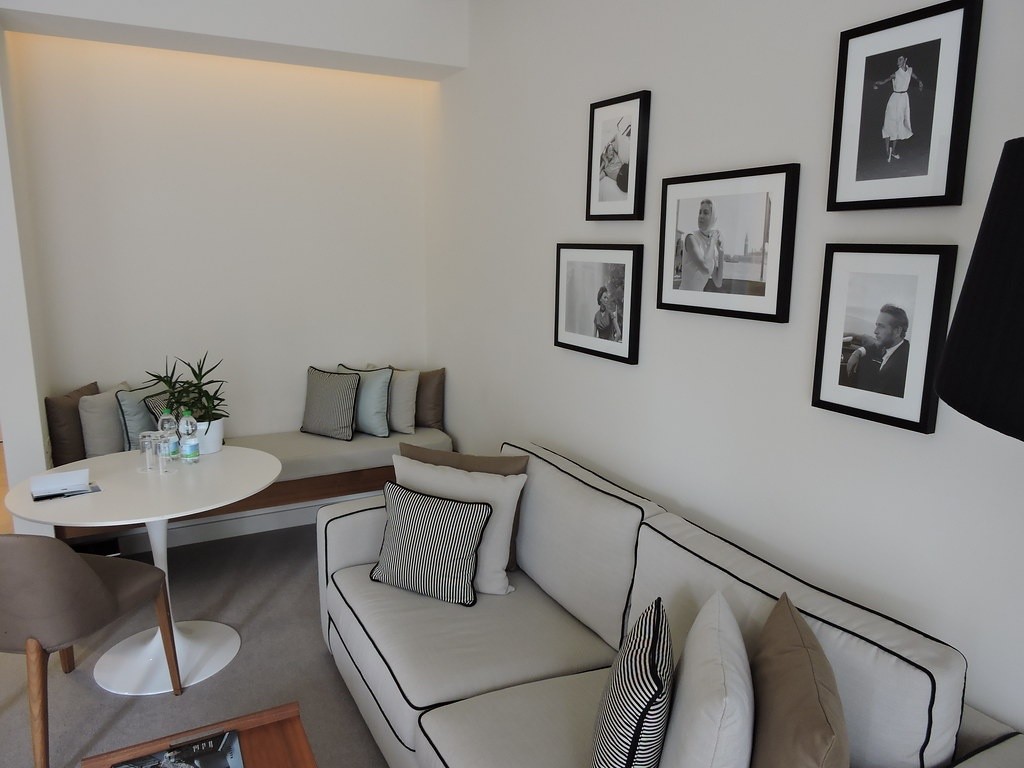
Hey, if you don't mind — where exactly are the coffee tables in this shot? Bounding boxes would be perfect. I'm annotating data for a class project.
[80,700,319,768]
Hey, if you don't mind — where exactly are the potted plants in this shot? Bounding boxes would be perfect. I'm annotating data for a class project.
[135,350,230,455]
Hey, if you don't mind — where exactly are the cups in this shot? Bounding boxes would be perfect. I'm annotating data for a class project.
[140,431,175,474]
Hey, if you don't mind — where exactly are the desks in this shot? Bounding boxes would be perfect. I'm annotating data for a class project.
[3,445,282,696]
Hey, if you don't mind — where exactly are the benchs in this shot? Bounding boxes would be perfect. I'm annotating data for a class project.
[57,429,458,557]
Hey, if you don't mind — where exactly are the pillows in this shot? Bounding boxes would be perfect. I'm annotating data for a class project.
[658,590,754,768]
[114,381,167,452]
[299,364,361,442]
[749,590,851,768]
[393,453,527,596]
[369,480,493,607]
[143,383,226,445]
[44,381,100,468]
[398,441,531,572]
[589,596,675,768]
[337,363,394,439]
[367,364,420,435]
[78,381,132,459]
[388,364,446,433]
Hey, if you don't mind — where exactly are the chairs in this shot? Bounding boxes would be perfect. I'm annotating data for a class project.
[0,533,184,768]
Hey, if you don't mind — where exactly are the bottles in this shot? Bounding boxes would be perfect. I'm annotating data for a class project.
[157,410,180,460]
[179,411,200,465]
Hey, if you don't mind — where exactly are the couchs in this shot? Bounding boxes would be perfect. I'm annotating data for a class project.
[315,439,1024,768]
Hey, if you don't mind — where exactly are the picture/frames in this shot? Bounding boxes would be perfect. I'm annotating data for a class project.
[810,243,959,435]
[554,241,644,365]
[585,90,651,221]
[826,0,984,212]
[656,162,801,324]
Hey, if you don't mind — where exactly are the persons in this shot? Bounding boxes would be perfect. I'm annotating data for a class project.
[601,144,628,192]
[872,51,923,162]
[846,304,911,398]
[592,286,621,342]
[677,198,727,293]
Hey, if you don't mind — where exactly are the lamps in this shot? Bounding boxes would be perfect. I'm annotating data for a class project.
[932,137,1024,443]
